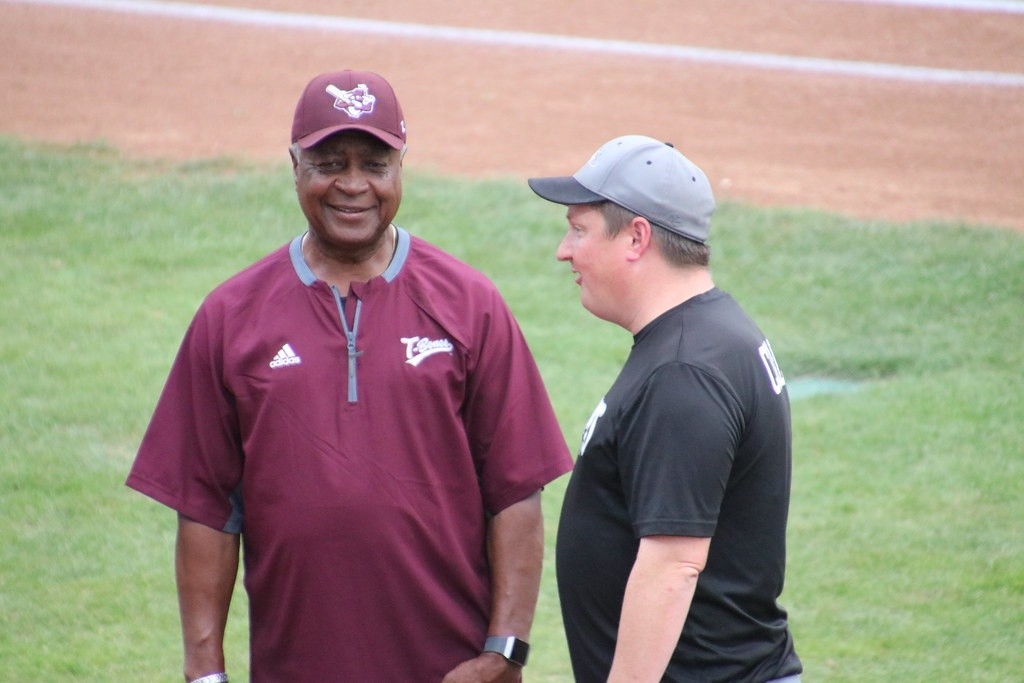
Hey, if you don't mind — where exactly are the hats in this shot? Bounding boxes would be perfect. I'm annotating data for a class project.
[291,68,407,151]
[527,135,715,243]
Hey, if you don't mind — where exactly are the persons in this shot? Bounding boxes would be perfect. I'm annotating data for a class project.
[124,68,575,683]
[528,134,806,683]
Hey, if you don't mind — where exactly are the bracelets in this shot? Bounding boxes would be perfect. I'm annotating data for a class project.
[189,672,228,683]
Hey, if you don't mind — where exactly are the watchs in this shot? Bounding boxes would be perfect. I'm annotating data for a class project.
[483,635,529,666]
